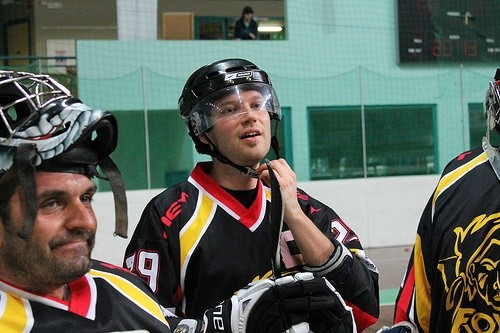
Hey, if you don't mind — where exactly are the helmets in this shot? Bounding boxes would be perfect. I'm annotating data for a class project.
[483,69,500,132]
[0,70,118,176]
[178,59,282,153]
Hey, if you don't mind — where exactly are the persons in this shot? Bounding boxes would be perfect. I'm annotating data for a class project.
[376,67,500,333]
[123,58,380,333]
[233,6,259,41]
[0,69,179,333]
